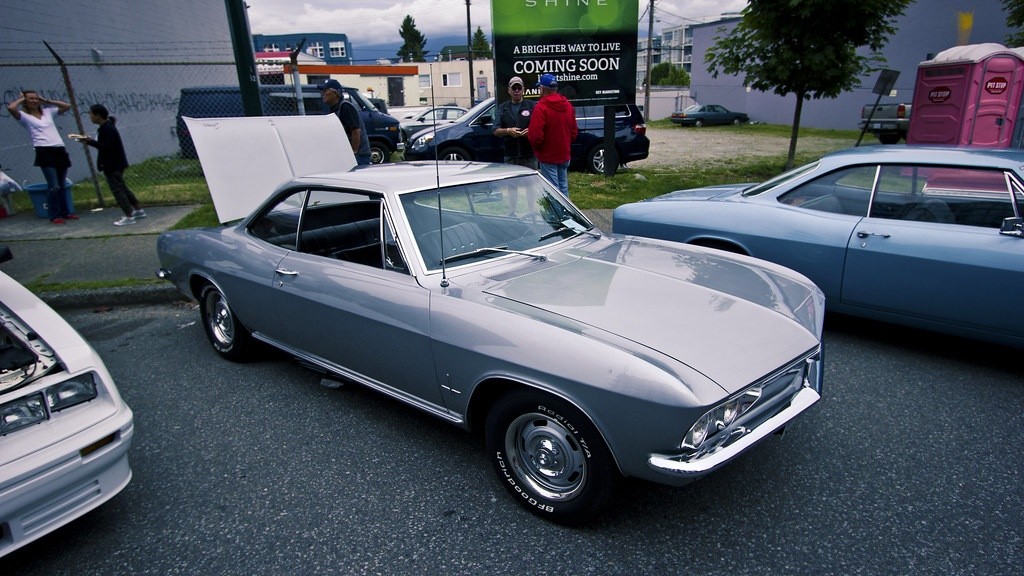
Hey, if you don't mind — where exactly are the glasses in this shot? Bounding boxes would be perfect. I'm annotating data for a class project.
[511,86,523,91]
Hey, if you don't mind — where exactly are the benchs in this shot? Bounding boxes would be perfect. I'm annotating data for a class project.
[796,195,842,214]
[269,218,488,271]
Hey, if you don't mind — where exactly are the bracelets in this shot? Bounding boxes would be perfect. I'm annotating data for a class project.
[47,99,48,102]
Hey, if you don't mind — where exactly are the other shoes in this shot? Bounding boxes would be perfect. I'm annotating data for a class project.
[64,213,81,220]
[49,216,66,224]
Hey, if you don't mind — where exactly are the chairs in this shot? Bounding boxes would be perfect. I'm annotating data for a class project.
[907,199,955,225]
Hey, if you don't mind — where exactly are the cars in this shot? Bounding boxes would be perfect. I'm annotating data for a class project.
[612,144,1024,347]
[0,245,134,559]
[398,106,492,143]
[670,104,750,127]
[157,112,827,523]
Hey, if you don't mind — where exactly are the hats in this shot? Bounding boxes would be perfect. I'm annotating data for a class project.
[317,79,343,93]
[508,76,524,88]
[535,74,559,89]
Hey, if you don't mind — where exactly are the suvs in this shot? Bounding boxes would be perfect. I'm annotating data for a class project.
[401,96,651,175]
[175,85,405,165]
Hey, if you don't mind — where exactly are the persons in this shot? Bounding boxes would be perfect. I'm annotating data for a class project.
[317,79,371,165]
[493,77,536,216]
[76,104,146,226]
[529,74,578,217]
[8,90,79,224]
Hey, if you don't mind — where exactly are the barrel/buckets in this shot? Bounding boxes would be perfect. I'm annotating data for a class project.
[24,182,74,218]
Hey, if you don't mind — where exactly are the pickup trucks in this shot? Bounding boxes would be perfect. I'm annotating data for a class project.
[859,103,912,144]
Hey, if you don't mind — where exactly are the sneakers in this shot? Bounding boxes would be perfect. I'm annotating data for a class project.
[131,209,148,218]
[113,215,138,225]
